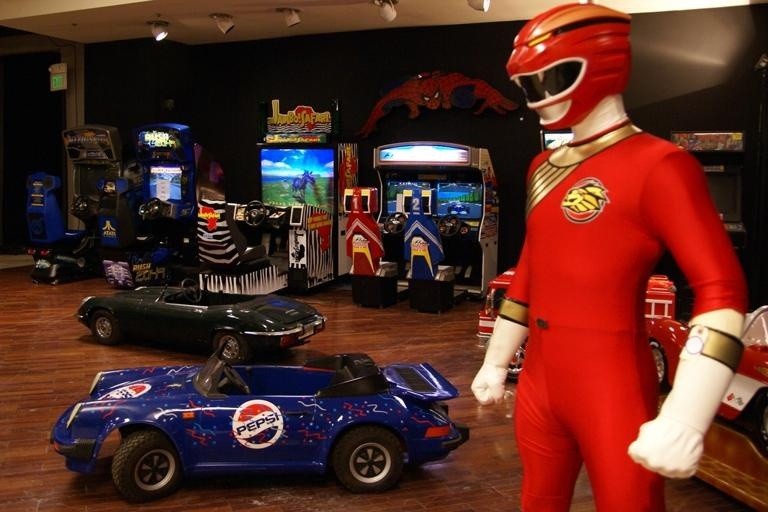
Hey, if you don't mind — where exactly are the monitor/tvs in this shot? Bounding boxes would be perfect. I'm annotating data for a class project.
[143,162,183,203]
[385,180,432,217]
[72,163,110,200]
[256,142,336,214]
[434,181,482,220]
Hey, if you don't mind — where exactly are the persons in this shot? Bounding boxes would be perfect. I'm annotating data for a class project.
[471,3,749,512]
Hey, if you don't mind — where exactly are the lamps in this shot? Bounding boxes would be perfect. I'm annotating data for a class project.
[282,10,302,29]
[466,1,491,14]
[149,21,169,40]
[211,15,235,35]
[379,1,398,23]
